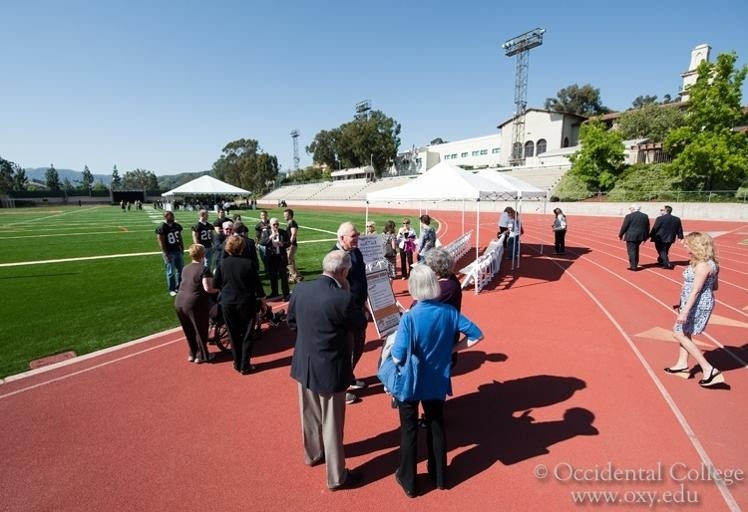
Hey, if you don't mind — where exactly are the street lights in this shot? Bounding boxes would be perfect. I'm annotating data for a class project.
[290,128,301,185]
[355,99,372,178]
[501,28,546,167]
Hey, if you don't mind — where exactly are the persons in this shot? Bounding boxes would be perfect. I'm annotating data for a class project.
[120,195,486,497]
[552,208,567,254]
[499,206,514,248]
[617,204,650,271]
[497,210,519,261]
[663,232,724,386]
[649,205,684,269]
[79,199,82,206]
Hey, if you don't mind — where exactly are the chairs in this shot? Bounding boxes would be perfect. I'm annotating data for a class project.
[407,224,505,295]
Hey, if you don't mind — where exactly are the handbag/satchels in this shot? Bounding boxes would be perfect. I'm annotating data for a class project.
[377,313,421,403]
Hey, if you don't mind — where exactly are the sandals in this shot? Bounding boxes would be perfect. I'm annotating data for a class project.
[698,367,725,386]
[663,366,692,380]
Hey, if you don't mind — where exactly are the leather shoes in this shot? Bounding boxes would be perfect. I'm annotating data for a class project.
[240,365,256,374]
[395,467,416,497]
[334,466,362,489]
[282,295,291,302]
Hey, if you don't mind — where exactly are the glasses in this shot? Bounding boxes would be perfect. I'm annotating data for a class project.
[274,223,279,226]
[226,228,232,230]
[403,223,408,224]
[351,232,360,237]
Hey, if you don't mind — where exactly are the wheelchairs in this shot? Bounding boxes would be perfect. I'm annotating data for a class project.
[205,283,282,356]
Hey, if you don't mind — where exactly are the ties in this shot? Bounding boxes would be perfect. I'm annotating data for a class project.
[273,236,276,255]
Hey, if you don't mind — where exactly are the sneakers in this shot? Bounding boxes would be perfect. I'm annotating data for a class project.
[195,352,216,362]
[344,392,356,405]
[349,378,365,390]
[269,320,287,328]
[272,309,285,319]
